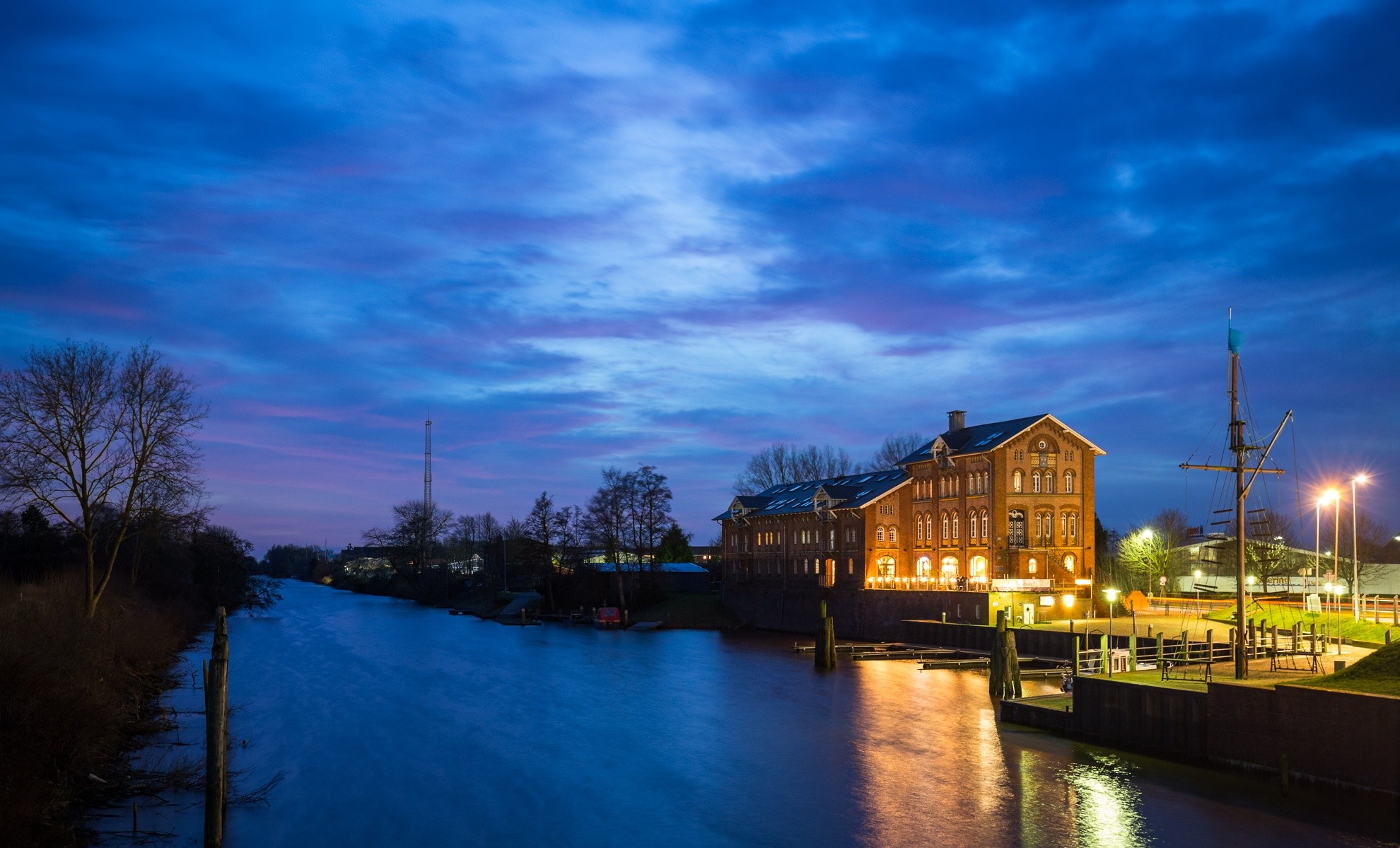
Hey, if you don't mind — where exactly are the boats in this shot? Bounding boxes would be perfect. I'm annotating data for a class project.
[1060,610,1174,693]
[591,607,621,630]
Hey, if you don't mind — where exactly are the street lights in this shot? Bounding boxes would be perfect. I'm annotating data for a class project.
[1194,570,1201,620]
[1247,575,1255,619]
[1314,471,1367,625]
[1143,528,1153,597]
[1106,588,1117,677]
